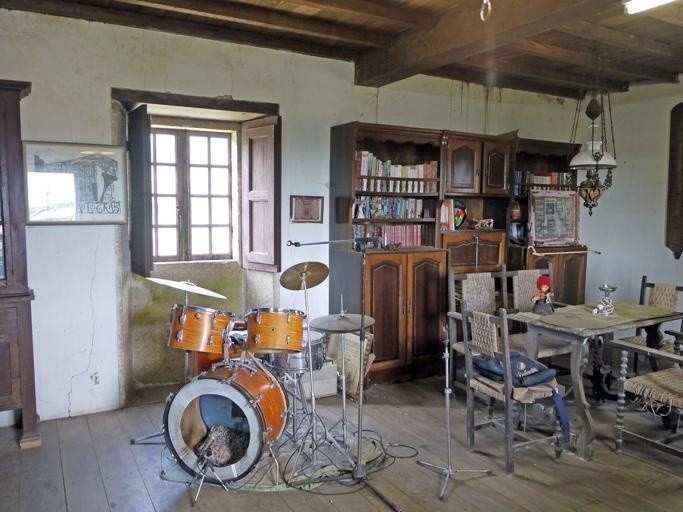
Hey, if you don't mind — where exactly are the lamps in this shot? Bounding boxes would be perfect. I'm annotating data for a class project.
[564,87,619,218]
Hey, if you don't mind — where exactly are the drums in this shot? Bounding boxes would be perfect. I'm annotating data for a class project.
[267,326,326,371]
[183,322,246,386]
[162,360,289,483]
[247,309,304,355]
[167,304,233,354]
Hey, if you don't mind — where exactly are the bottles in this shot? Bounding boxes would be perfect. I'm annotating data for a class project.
[510,198,522,219]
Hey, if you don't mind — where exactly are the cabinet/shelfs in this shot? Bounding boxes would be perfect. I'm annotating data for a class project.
[524,249,587,304]
[446,132,513,229]
[445,234,504,269]
[512,146,578,246]
[355,136,441,251]
[365,252,444,370]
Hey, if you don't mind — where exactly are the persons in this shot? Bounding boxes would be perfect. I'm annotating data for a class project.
[530,276,556,315]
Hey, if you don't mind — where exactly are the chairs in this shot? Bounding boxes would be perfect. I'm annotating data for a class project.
[450,267,600,405]
[607,275,682,403]
[610,330,683,459]
[456,299,561,473]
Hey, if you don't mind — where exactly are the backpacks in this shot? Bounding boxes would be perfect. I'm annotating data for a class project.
[474,350,571,442]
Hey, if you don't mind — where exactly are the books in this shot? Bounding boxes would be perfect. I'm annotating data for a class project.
[515,168,572,195]
[444,197,455,232]
[355,150,439,248]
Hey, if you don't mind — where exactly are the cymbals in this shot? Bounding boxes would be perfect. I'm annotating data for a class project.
[146,277,227,298]
[310,314,376,331]
[280,262,330,290]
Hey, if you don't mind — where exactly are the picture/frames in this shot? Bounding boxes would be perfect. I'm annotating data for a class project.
[290,196,324,223]
